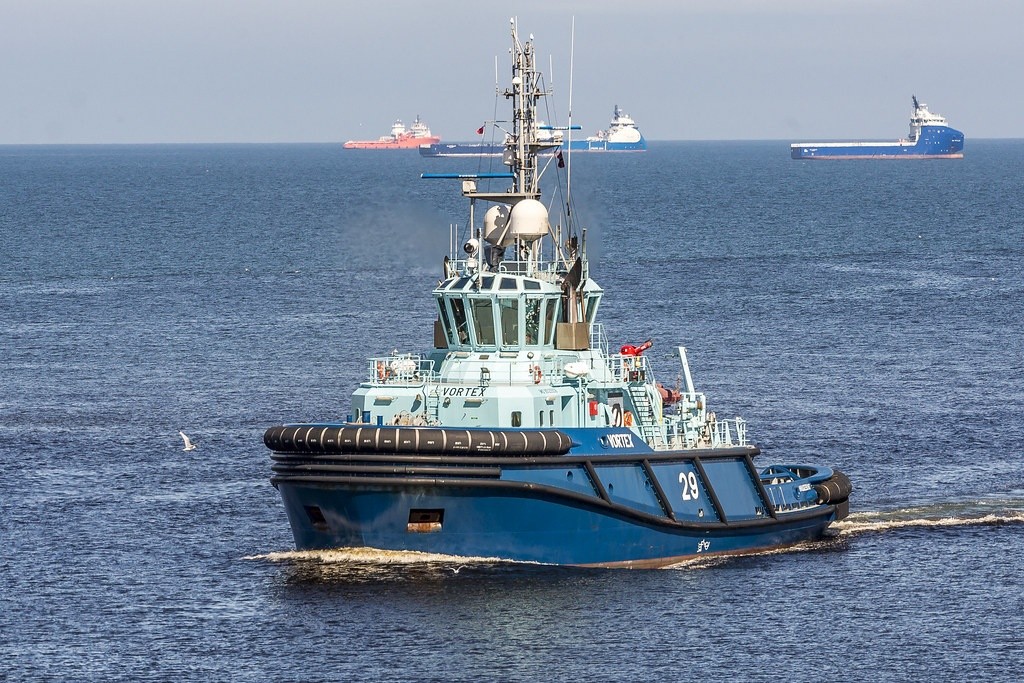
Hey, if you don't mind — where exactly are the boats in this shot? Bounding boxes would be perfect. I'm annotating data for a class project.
[262,15,853,569]
[418,104,648,158]
[342,114,440,151]
[791,93,965,159]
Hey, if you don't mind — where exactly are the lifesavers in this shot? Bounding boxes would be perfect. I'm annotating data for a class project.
[623,410,633,427]
[534,366,541,385]
